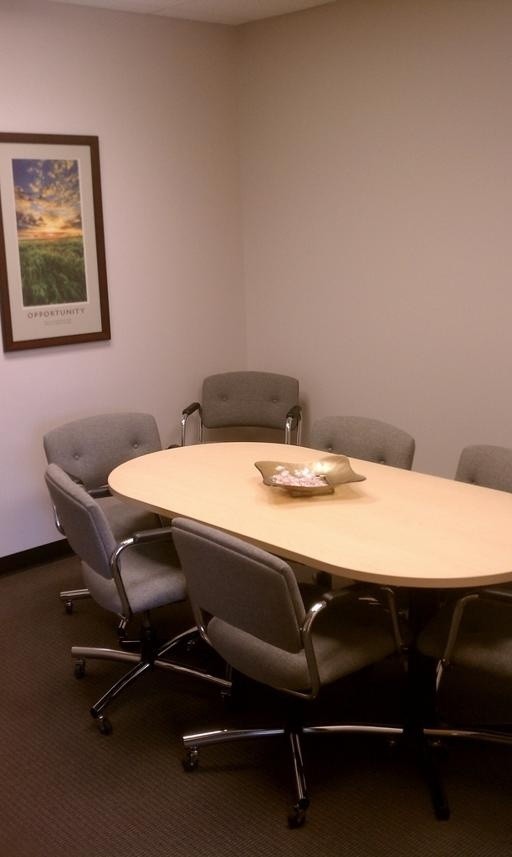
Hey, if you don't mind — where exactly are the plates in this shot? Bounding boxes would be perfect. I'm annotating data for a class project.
[254,454,368,498]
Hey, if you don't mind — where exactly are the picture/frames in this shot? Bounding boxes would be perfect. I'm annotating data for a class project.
[1,131,113,354]
